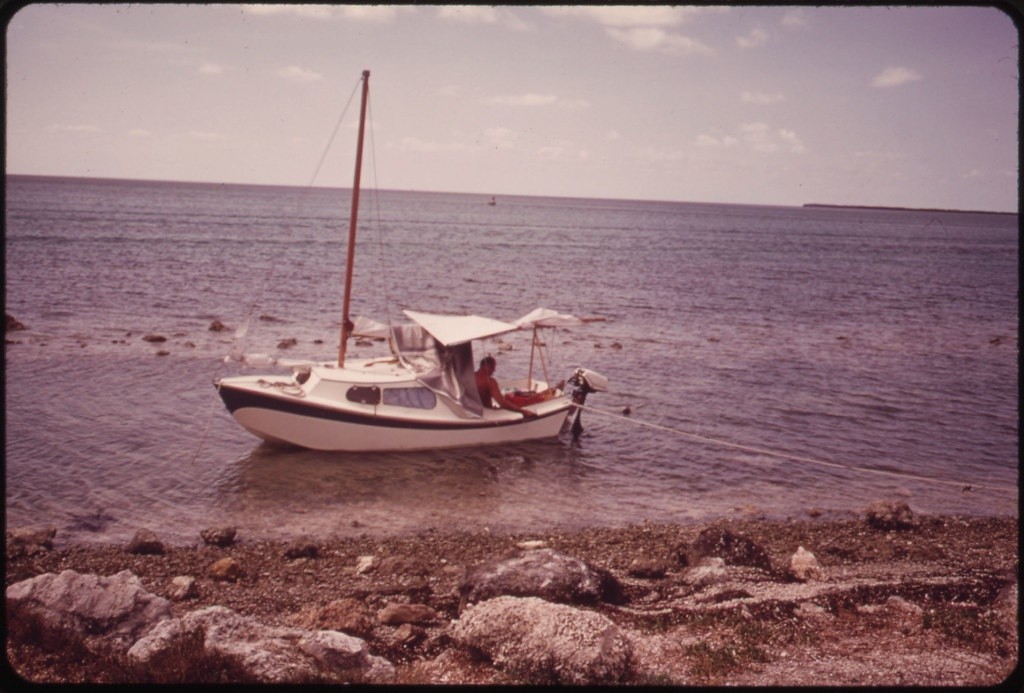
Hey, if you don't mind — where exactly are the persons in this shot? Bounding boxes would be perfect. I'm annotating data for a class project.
[474,356,537,419]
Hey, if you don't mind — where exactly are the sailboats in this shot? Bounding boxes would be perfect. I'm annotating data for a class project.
[211,69,572,448]
